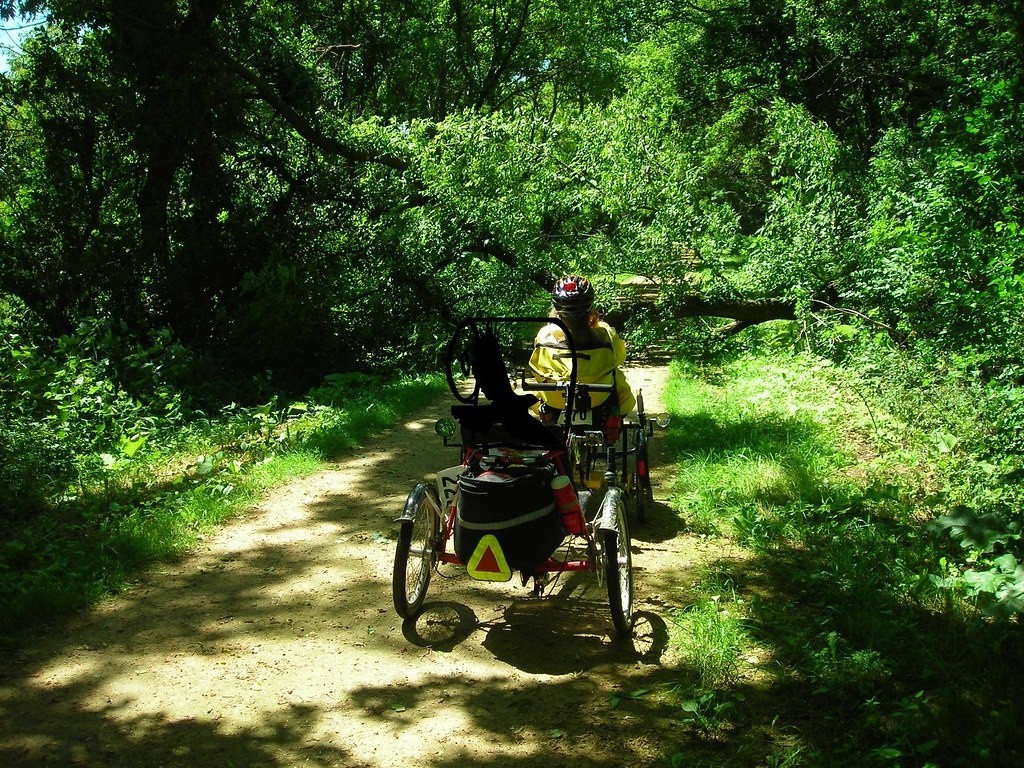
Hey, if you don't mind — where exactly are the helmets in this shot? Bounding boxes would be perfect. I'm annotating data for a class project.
[551,276,594,318]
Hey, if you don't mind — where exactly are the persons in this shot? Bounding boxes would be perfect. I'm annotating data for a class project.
[529,274,639,425]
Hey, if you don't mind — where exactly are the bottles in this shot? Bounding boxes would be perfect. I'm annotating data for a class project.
[553,476,587,535]
